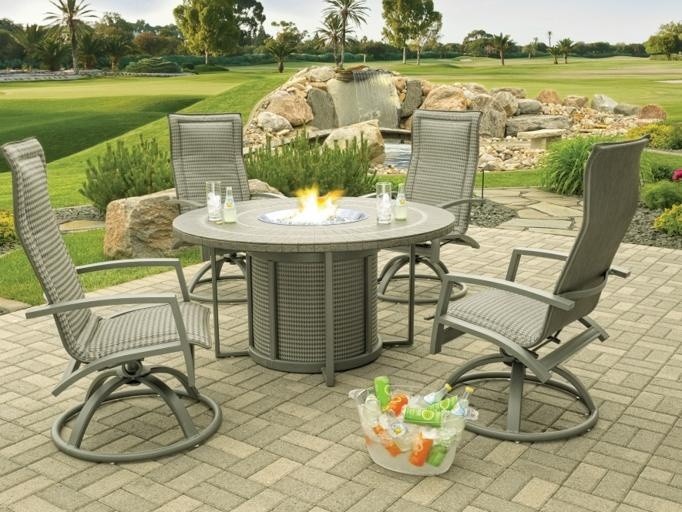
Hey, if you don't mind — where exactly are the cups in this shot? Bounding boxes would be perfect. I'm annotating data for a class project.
[206,181,223,221]
[376,181,392,224]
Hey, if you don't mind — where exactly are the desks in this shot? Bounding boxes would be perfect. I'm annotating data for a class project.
[173,195,457,387]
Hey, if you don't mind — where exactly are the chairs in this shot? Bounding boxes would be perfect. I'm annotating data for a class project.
[430,126,650,446]
[168,112,286,303]
[2,137,225,463]
[358,108,487,305]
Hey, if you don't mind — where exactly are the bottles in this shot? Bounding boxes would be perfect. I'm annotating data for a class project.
[361,376,473,467]
[395,184,408,221]
[223,186,236,224]
